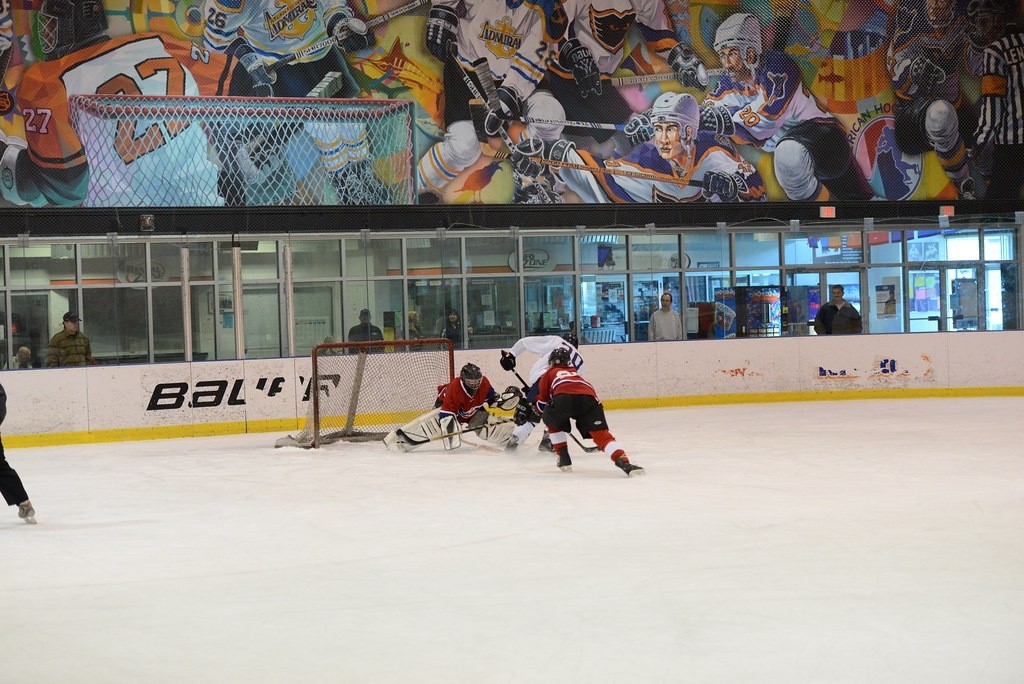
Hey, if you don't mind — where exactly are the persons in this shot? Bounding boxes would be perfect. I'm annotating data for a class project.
[3,346,32,369]
[401,311,424,350]
[0,384,38,526]
[383,332,646,477]
[348,308,385,353]
[317,335,340,356]
[440,309,473,350]
[48,310,91,367]
[648,292,687,341]
[569,321,589,343]
[814,285,862,335]
[885,290,895,304]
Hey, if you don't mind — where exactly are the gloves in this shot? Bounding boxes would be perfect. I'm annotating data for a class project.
[500,352,517,372]
[514,398,529,426]
[526,406,540,424]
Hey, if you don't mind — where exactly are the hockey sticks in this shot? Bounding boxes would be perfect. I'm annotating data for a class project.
[511,367,602,453]
[461,438,540,456]
[395,417,516,446]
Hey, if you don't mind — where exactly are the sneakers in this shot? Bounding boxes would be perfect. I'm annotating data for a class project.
[507,436,518,455]
[615,459,646,478]
[555,445,572,474]
[539,430,555,452]
[19,500,37,524]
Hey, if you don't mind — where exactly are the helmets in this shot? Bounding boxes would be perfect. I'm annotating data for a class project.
[549,348,571,368]
[460,363,482,390]
[562,332,579,349]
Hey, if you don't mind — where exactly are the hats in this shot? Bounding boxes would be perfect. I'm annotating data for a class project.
[360,309,371,314]
[63,312,83,322]
